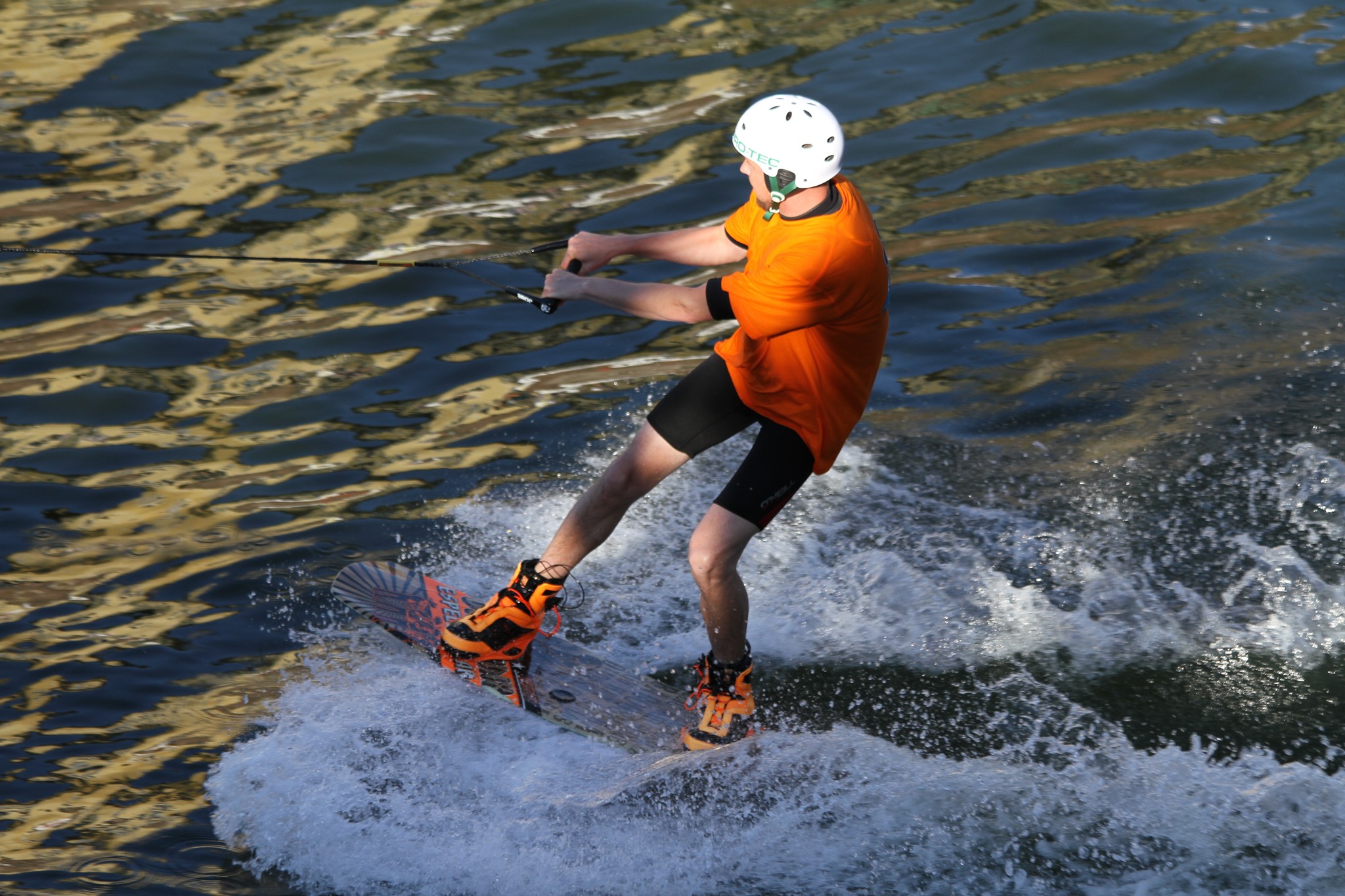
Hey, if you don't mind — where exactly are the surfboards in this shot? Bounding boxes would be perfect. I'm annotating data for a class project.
[331,555,762,765]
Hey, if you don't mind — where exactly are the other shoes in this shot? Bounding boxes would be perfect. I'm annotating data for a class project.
[682,649,757,751]
[441,557,562,660]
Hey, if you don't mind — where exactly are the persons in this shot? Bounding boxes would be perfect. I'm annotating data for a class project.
[438,93,889,752]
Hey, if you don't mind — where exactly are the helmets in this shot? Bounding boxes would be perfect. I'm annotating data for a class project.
[733,94,843,188]
